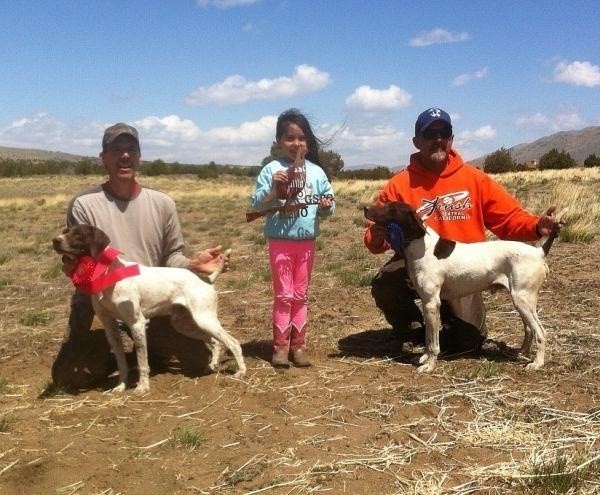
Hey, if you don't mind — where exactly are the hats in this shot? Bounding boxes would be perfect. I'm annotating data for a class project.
[415,108,452,137]
[102,123,139,146]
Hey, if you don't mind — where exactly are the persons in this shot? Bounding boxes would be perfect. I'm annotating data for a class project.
[249,109,336,368]
[363,108,568,353]
[62,122,231,375]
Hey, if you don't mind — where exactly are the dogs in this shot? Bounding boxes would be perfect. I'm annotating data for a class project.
[364,201,569,374]
[51,224,247,395]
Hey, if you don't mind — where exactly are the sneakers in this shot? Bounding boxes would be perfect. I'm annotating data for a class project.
[271,347,290,368]
[289,345,310,367]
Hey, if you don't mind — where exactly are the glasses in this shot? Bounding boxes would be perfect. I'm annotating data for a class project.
[420,127,452,139]
